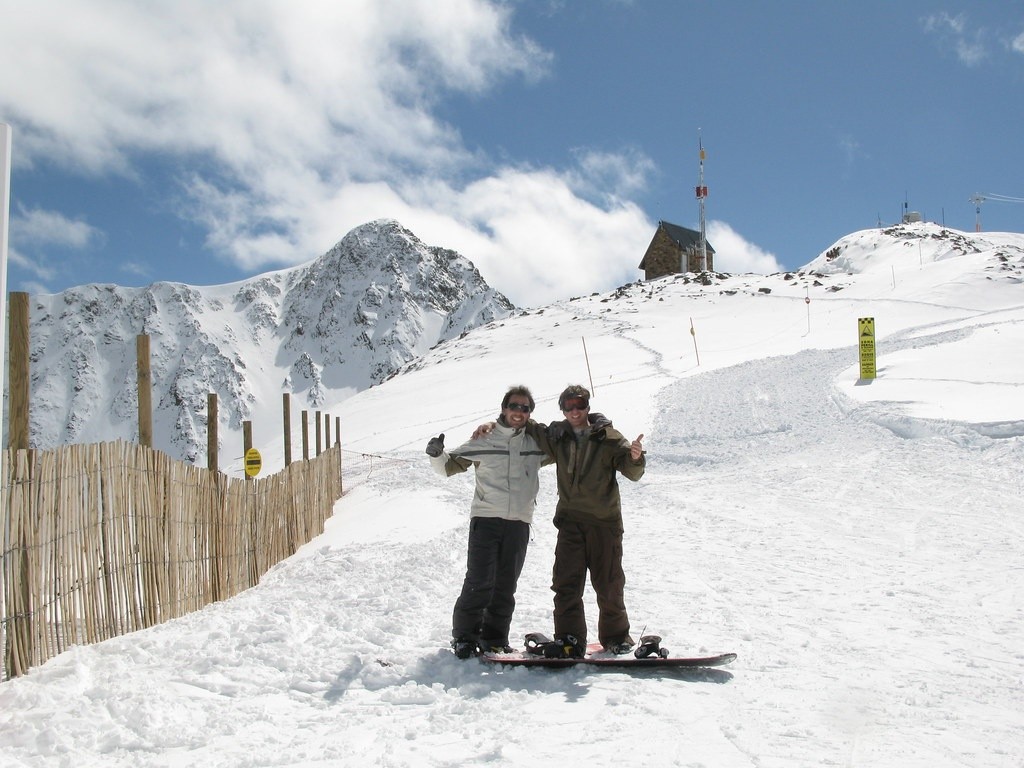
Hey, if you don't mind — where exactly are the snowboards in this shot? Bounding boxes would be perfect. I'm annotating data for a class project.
[482,641,739,670]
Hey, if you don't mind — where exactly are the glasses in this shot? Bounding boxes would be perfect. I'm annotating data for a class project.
[561,396,588,412]
[506,402,529,413]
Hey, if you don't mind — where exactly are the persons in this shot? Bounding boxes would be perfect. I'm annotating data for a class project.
[470,385,647,657]
[425,386,557,659]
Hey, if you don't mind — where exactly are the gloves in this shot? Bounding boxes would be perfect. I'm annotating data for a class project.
[426,433,445,457]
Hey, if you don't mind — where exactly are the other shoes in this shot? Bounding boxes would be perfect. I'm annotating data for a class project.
[450,637,482,658]
[602,640,631,654]
[544,638,586,659]
[480,639,514,653]
